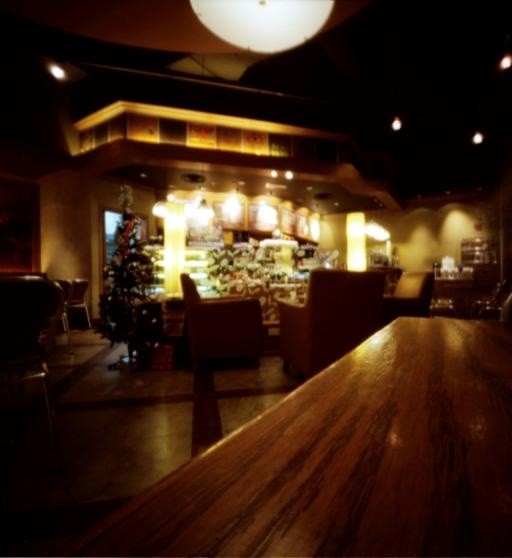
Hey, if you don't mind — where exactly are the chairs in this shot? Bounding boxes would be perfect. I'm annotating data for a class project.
[1,276,90,416]
[276,263,434,379]
[180,269,266,369]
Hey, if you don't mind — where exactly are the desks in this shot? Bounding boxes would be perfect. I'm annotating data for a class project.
[50,316,511,551]
